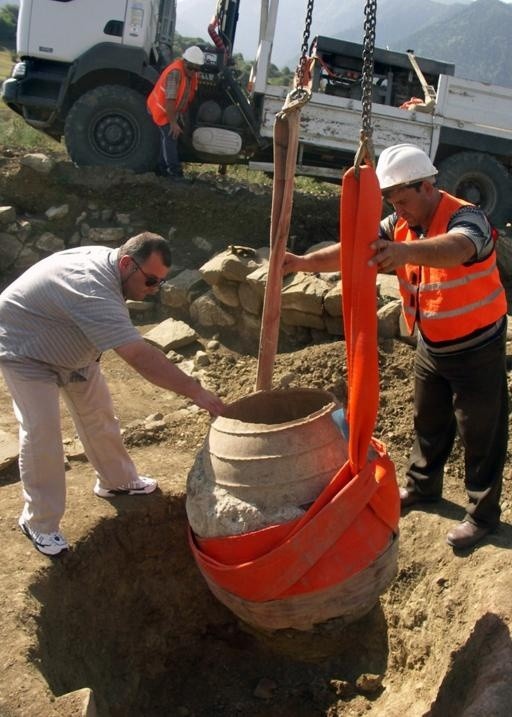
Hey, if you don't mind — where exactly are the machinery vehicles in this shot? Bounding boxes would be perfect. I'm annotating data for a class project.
[0,1,512,231]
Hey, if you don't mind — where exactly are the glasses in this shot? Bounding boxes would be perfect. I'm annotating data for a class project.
[187,64,200,72]
[131,257,165,287]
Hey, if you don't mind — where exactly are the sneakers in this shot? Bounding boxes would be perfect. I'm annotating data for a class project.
[18,515,69,558]
[94,476,158,498]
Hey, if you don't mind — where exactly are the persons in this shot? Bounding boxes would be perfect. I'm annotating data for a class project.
[0,232,224,556]
[279,143,509,549]
[145,45,205,182]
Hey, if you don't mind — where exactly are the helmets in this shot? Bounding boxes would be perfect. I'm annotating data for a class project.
[182,46,205,65]
[376,143,439,190]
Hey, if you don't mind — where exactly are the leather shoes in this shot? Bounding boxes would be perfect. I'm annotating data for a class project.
[399,487,442,507]
[447,521,491,550]
